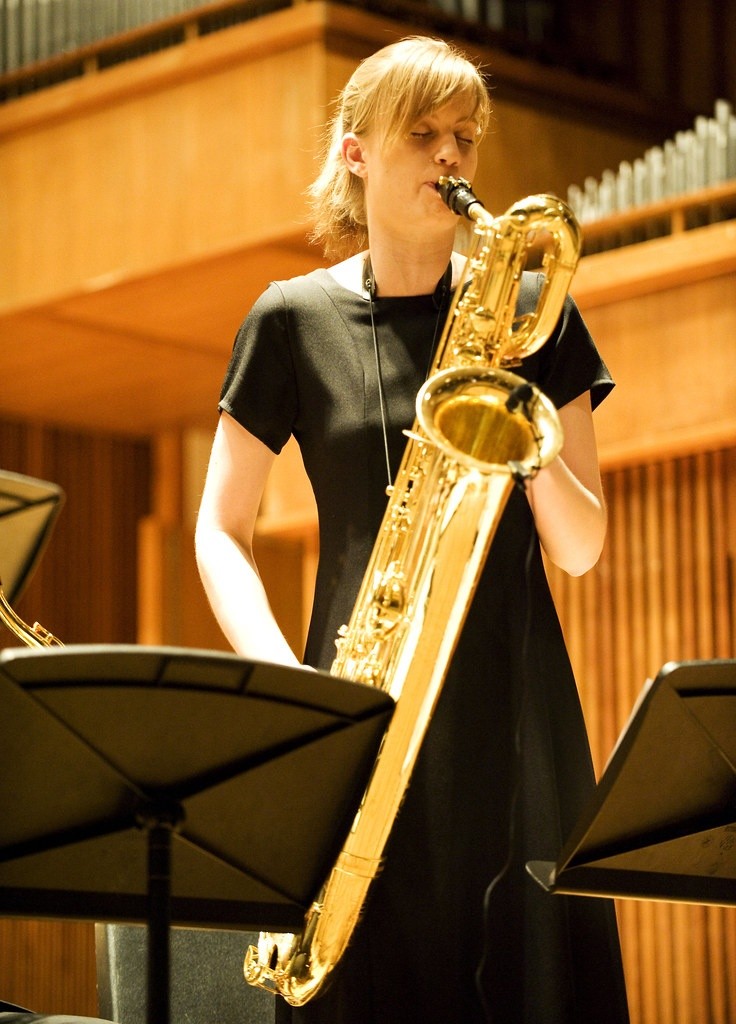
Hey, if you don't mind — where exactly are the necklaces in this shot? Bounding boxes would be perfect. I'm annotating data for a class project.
[366,273,448,487]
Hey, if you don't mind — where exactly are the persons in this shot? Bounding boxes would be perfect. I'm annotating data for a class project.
[193,36,631,1024]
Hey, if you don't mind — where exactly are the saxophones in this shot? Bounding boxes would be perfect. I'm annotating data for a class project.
[222,175,584,1008]
